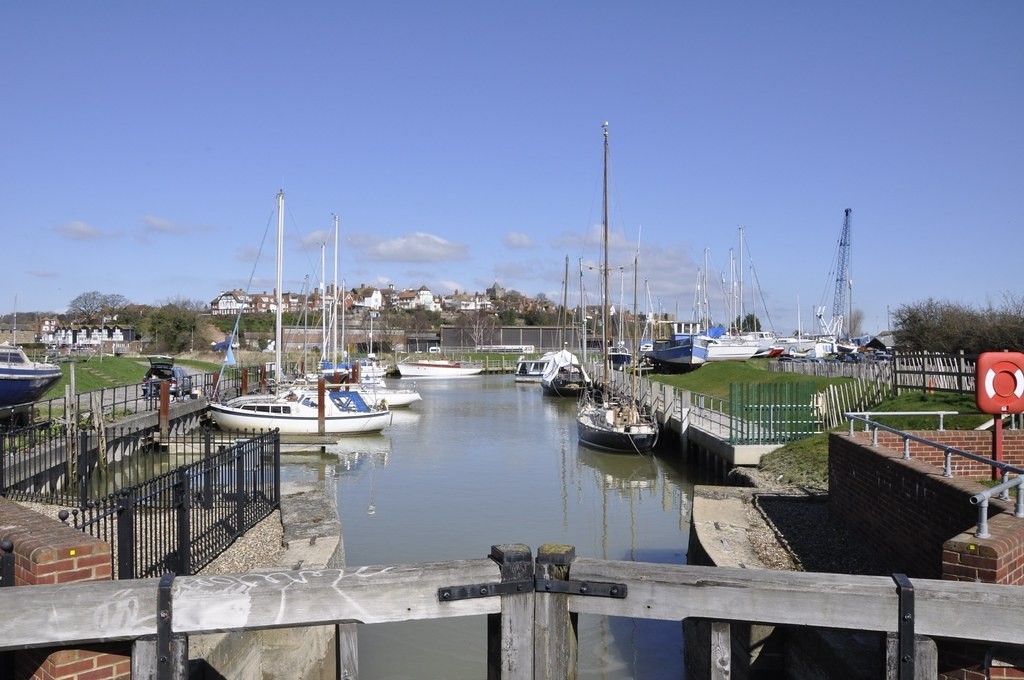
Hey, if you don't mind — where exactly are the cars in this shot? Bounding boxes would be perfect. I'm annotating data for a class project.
[869,355,894,365]
[429,347,440,353]
[142,355,193,397]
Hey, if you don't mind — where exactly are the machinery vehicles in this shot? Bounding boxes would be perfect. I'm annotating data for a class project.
[816,208,853,344]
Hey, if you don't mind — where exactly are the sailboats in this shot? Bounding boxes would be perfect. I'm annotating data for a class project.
[608,271,632,370]
[578,445,659,562]
[575,119,660,455]
[541,253,594,398]
[205,188,392,434]
[638,227,818,372]
[279,212,423,407]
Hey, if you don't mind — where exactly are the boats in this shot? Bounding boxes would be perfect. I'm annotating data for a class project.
[396,360,483,377]
[515,352,558,383]
[0,341,63,421]
